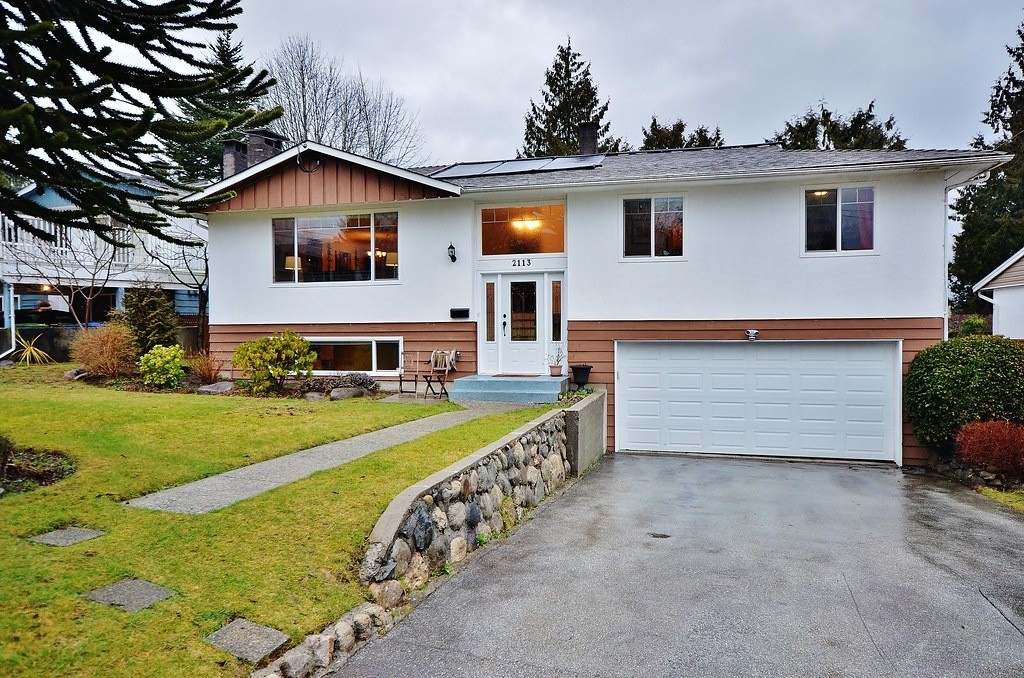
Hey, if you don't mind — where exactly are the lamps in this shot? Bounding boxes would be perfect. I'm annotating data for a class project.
[386,252,398,278]
[448,241,457,262]
[285,256,302,281]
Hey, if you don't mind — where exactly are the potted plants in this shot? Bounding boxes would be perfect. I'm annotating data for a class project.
[569,363,593,392]
[541,335,568,376]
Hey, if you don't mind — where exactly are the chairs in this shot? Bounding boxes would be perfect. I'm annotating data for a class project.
[422,351,451,399]
[399,351,419,398]
